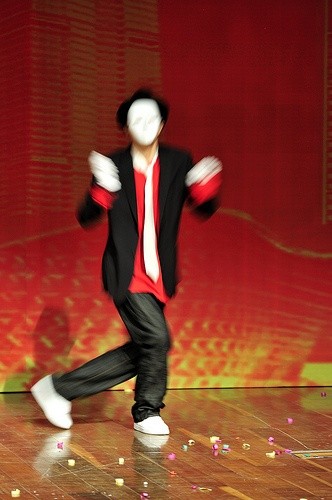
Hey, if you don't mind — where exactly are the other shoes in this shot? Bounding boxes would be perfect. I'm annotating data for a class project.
[30,373,74,430]
[133,416,169,437]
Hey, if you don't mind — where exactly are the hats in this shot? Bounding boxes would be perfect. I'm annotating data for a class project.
[116,88,169,129]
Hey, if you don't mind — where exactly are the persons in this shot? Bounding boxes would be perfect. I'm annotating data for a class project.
[30,88,221,436]
